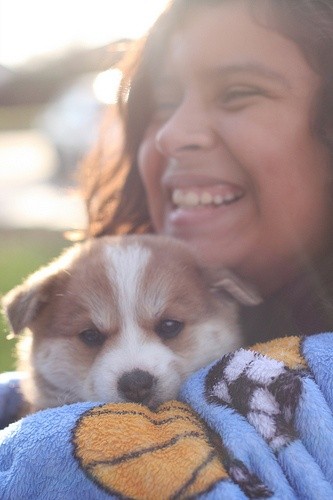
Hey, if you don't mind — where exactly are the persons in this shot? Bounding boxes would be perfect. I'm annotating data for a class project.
[0,1,332,345]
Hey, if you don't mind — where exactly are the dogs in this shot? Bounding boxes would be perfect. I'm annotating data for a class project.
[2,233,264,422]
